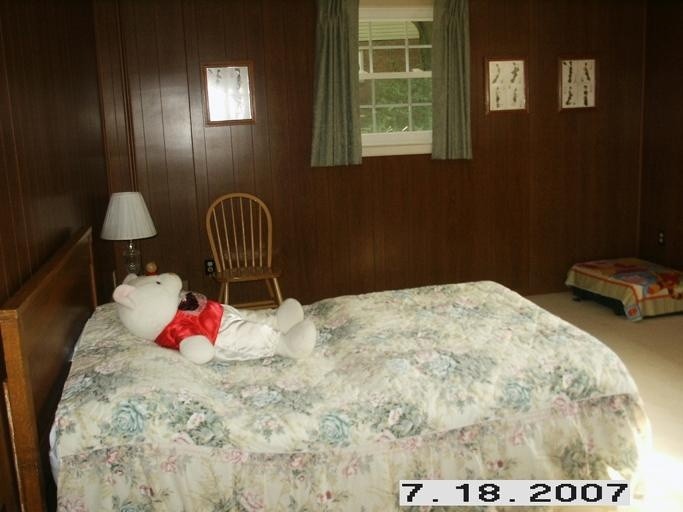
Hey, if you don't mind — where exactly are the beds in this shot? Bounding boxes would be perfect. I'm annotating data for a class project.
[0,224,638,510]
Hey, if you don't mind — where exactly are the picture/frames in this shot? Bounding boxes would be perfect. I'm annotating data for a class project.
[197,60,257,127]
[482,53,601,116]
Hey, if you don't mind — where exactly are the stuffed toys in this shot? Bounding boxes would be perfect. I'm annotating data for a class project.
[110,272,316,362]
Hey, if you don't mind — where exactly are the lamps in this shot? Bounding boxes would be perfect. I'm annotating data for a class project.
[99,191,157,275]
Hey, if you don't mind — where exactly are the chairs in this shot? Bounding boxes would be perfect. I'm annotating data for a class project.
[204,192,283,310]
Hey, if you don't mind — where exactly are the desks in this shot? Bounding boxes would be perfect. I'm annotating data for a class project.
[571,257,682,316]
